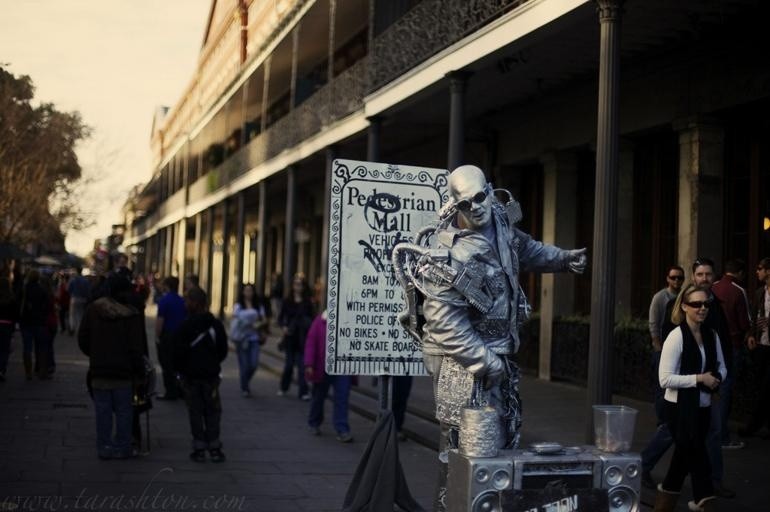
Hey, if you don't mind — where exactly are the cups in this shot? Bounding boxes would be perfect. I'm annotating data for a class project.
[458,405,499,459]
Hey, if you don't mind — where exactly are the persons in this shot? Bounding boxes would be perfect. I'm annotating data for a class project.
[392,164,587,512]
[640,257,770,512]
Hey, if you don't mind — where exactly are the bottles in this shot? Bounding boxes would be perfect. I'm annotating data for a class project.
[757,309,762,319]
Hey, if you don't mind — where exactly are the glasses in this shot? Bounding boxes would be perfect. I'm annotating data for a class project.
[680,299,712,309]
[757,267,765,271]
[668,274,684,280]
[453,186,490,213]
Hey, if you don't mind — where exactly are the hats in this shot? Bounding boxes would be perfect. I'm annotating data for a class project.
[164,276,178,291]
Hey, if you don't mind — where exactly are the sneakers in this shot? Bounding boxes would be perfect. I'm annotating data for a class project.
[641,470,718,511]
[300,395,312,401]
[242,391,252,399]
[157,393,177,401]
[189,450,226,463]
[95,446,138,460]
[275,390,287,397]
[305,424,321,437]
[335,431,353,444]
[721,439,745,451]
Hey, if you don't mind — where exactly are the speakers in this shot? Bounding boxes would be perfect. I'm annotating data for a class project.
[444,447,513,512]
[583,444,642,512]
